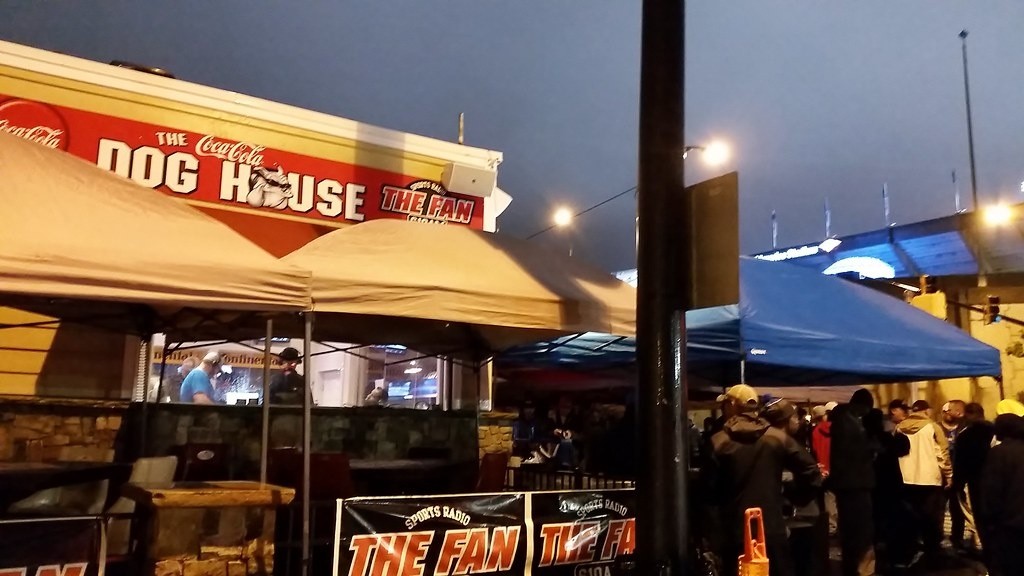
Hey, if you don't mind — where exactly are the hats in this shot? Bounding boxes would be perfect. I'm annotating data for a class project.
[278,347,302,364]
[715,383,759,407]
[889,399,911,410]
[825,401,839,411]
[912,400,934,412]
[997,398,1024,417]
[758,397,798,424]
[810,405,826,419]
[852,388,873,413]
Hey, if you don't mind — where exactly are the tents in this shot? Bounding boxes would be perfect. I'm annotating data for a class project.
[0,131,638,576]
[501,254,1003,404]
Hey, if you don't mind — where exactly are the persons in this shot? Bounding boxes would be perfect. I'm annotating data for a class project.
[158,346,1024,576]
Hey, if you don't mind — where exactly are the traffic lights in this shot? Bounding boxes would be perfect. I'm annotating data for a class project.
[920,274,936,295]
[983,294,1000,325]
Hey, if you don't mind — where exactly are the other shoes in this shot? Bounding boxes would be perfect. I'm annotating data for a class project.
[957,544,968,554]
